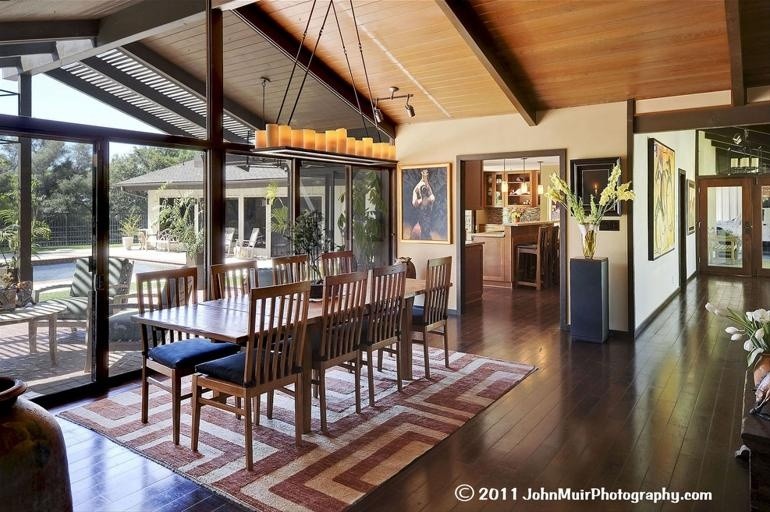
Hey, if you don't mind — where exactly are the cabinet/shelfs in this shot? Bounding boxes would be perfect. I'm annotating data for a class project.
[504,168,535,210]
[530,168,543,210]
[465,159,485,211]
[483,170,504,208]
[472,233,510,291]
[477,222,507,233]
[465,240,485,311]
[736,354,770,512]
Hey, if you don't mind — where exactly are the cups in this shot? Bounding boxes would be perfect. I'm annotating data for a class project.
[487,175,532,205]
[465,209,474,233]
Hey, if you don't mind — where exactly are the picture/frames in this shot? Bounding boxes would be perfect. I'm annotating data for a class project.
[685,177,698,236]
[395,162,454,247]
[646,136,676,261]
[567,156,624,218]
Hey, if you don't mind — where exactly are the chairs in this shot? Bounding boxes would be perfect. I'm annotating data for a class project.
[710,226,739,264]
[0,243,464,476]
[513,222,563,290]
[142,225,261,263]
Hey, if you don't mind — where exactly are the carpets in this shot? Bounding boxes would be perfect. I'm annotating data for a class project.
[54,331,537,512]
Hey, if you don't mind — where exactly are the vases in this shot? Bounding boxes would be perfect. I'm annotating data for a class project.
[0,375,74,512]
[514,216,521,224]
[577,221,599,259]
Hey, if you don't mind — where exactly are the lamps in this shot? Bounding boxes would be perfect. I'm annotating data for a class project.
[248,0,399,176]
[369,82,417,124]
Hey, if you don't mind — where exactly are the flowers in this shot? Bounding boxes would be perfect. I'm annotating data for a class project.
[507,205,532,222]
[542,155,637,257]
[697,295,770,369]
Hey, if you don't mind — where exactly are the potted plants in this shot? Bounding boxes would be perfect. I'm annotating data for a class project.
[286,206,354,300]
[116,207,142,250]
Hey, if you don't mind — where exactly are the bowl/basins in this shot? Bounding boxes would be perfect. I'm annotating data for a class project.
[303,280,340,299]
